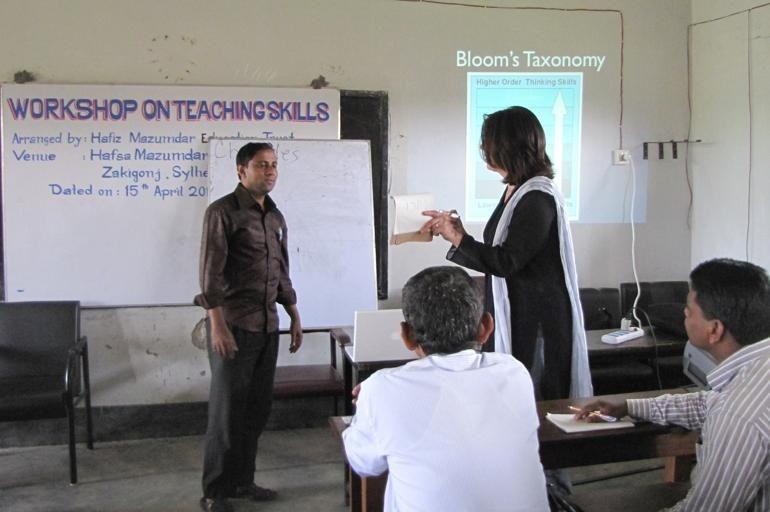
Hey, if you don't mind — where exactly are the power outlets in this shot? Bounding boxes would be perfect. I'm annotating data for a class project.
[612,150,631,165]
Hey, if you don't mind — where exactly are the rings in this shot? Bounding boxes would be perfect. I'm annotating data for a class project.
[436,223,439,228]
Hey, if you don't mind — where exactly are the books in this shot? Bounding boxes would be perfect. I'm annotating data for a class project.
[544,410,635,435]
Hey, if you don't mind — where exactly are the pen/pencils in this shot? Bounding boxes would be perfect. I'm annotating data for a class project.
[438,210,460,219]
[567,405,617,423]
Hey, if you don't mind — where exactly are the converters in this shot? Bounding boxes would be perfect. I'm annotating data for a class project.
[621,318,631,330]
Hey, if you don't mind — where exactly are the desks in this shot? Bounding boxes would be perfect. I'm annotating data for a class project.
[341,325,687,411]
[330,329,352,418]
[329,385,698,512]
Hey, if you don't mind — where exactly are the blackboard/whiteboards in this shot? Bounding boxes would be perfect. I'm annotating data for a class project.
[205,135,381,334]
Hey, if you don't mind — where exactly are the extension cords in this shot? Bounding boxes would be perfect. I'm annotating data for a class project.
[600,326,645,345]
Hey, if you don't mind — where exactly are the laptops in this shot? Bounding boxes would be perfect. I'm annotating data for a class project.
[344,308,420,362]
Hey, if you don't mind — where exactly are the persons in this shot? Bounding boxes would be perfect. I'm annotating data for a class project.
[574,259,770,511]
[341,265,552,512]
[418,106,596,497]
[193,142,303,511]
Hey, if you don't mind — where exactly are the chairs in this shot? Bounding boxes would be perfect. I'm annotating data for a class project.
[620,281,688,389]
[0,301,92,484]
[271,328,344,416]
[579,288,654,393]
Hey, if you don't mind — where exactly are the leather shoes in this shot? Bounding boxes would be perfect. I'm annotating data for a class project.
[232,484,278,502]
[200,495,237,512]
